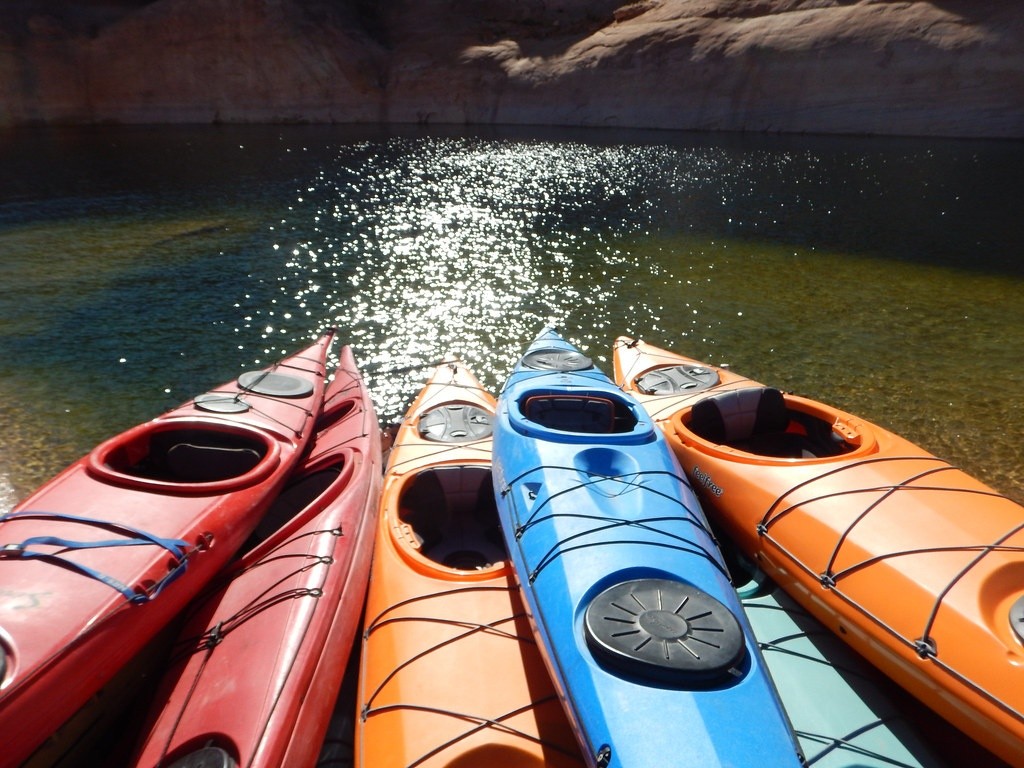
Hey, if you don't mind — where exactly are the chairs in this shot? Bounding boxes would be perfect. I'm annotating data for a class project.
[413,464,507,568]
[526,394,615,433]
[254,467,340,535]
[691,385,819,460]
[168,444,260,481]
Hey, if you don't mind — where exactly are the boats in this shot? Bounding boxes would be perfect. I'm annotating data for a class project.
[611,334,1024,762]
[353,354,587,762]
[2,327,337,762]
[493,327,803,762]
[141,347,383,762]
